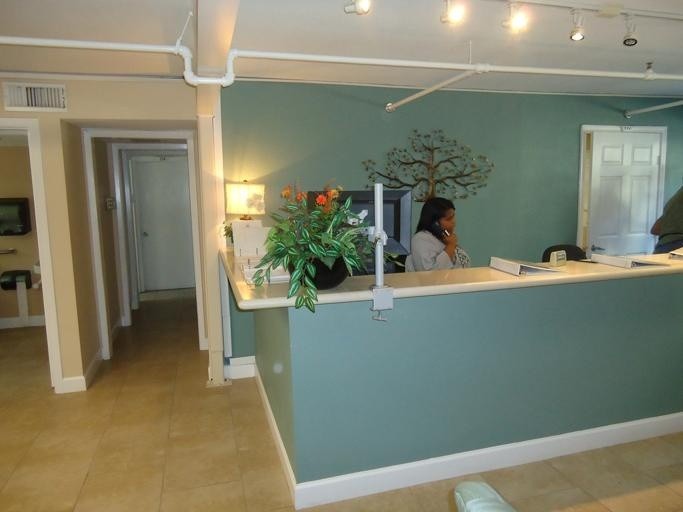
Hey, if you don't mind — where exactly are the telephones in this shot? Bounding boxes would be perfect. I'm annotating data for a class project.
[432,221,450,241]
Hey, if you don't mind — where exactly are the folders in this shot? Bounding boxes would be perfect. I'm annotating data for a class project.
[590,253,670,268]
[669,247,683,261]
[489,256,560,276]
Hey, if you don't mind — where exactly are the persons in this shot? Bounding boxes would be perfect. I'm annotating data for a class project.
[404,197,471,273]
[652,185,683,254]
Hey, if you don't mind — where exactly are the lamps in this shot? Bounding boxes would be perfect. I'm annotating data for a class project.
[438,1,465,23]
[344,0,370,15]
[500,2,637,46]
[226,180,266,220]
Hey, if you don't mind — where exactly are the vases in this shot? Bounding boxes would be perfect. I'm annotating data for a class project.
[287,256,349,289]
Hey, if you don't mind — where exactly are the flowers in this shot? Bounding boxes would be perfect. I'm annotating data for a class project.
[252,178,406,313]
